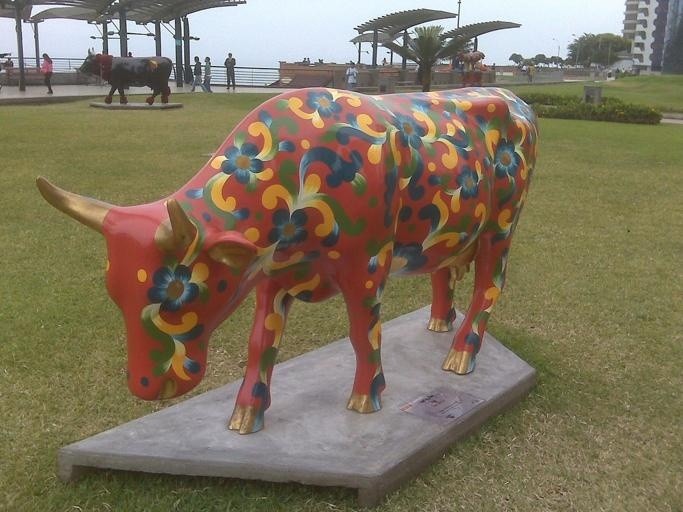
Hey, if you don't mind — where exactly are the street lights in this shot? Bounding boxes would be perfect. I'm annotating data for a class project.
[457,0,461,27]
[572,33,579,66]
[552,37,559,68]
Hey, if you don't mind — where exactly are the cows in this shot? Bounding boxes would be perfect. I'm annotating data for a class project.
[79,47,176,105]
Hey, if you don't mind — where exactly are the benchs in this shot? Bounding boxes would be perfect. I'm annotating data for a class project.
[5,66,55,88]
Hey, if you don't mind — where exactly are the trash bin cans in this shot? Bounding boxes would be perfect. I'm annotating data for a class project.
[584,86,602,105]
[378,77,395,94]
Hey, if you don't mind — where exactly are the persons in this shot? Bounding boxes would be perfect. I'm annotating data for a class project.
[521,63,632,83]
[189,55,207,94]
[201,58,212,93]
[40,52,55,95]
[222,53,237,90]
[345,62,358,91]
